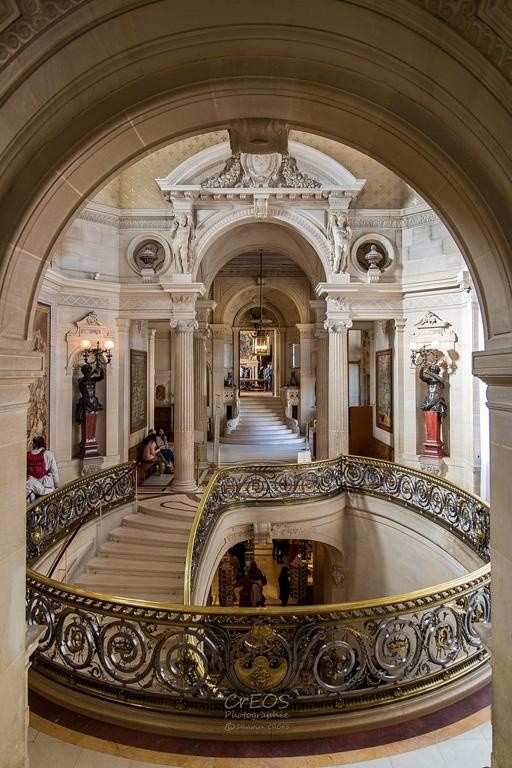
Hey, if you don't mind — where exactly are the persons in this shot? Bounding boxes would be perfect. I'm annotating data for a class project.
[148,428,175,468]
[169,213,191,275]
[223,539,317,607]
[329,213,353,275]
[74,362,107,428]
[141,433,174,474]
[240,363,273,392]
[27,435,60,497]
[155,427,169,449]
[415,362,447,415]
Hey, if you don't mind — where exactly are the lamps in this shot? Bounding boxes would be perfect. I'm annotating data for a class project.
[253,250,272,357]
[410,339,441,366]
[82,340,114,373]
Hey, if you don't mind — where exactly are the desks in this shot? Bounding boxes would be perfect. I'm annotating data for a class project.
[240,378,267,392]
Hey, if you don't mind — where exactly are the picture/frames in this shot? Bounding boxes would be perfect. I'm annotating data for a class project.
[129,349,147,434]
[26,301,52,455]
[375,349,393,432]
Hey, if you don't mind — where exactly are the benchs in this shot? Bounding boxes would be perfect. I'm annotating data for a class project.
[128,440,174,486]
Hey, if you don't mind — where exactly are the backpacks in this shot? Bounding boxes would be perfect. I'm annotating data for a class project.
[27,448,50,479]
